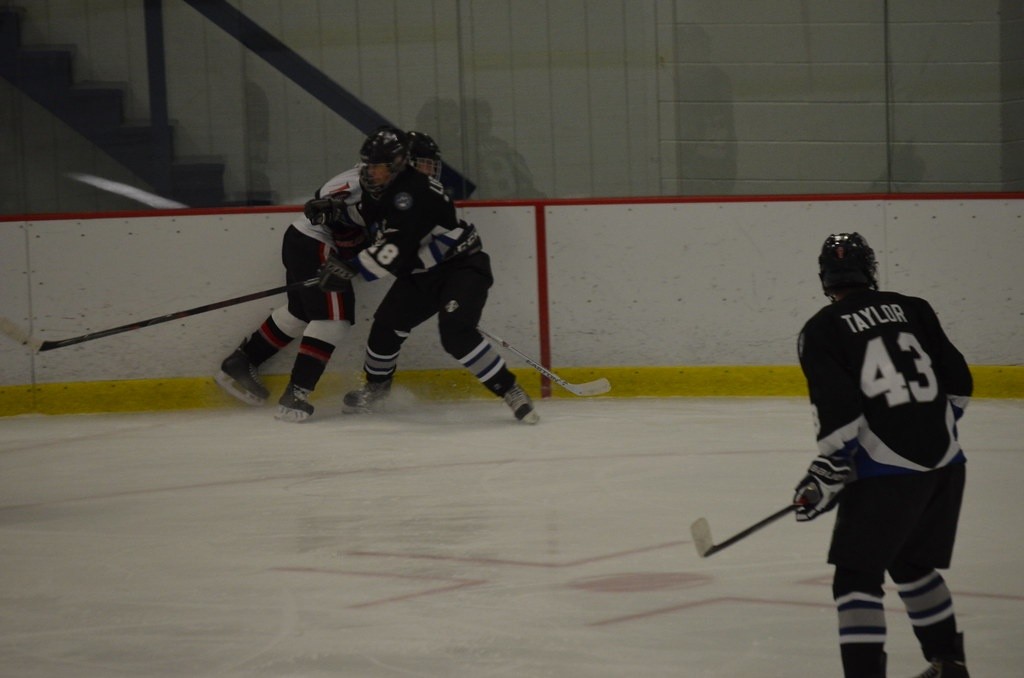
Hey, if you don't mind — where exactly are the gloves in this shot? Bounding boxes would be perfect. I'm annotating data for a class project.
[792,454,851,522]
[303,198,341,225]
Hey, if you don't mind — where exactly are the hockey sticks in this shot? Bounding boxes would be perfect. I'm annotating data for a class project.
[0,286,288,354]
[690,503,797,558]
[481,327,613,396]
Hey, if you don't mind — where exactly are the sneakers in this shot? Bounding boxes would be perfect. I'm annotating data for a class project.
[914,630,969,678]
[214,337,270,407]
[339,376,394,414]
[503,383,540,424]
[274,383,314,420]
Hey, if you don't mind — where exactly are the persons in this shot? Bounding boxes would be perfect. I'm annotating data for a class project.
[339,131,539,424]
[791,232,973,678]
[212,128,441,423]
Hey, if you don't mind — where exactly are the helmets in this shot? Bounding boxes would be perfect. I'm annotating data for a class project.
[358,126,408,193]
[817,231,876,287]
[406,131,443,181]
